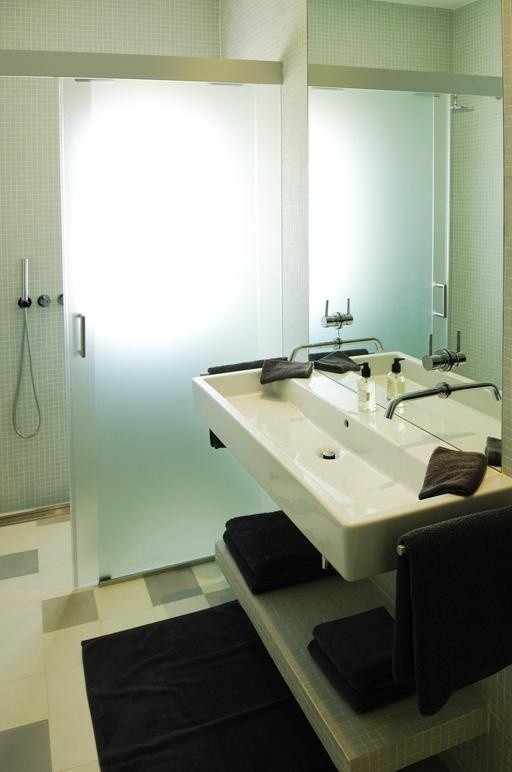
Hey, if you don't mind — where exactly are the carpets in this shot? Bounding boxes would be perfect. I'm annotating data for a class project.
[79,600,447,770]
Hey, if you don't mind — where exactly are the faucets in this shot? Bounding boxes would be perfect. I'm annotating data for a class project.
[383,383,446,419]
[445,381,502,401]
[289,338,340,364]
[340,337,384,351]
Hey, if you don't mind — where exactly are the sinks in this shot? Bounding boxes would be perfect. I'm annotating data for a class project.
[191,365,512,581]
[348,351,503,471]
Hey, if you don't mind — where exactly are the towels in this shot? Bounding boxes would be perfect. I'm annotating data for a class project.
[313,350,360,374]
[223,509,338,594]
[260,358,313,384]
[417,446,487,496]
[310,607,408,718]
[484,436,501,467]
[395,507,512,717]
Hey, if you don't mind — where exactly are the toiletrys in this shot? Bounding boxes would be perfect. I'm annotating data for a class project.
[357,361,377,414]
[387,357,407,415]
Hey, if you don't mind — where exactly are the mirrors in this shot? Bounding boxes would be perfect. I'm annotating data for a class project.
[306,1,504,475]
